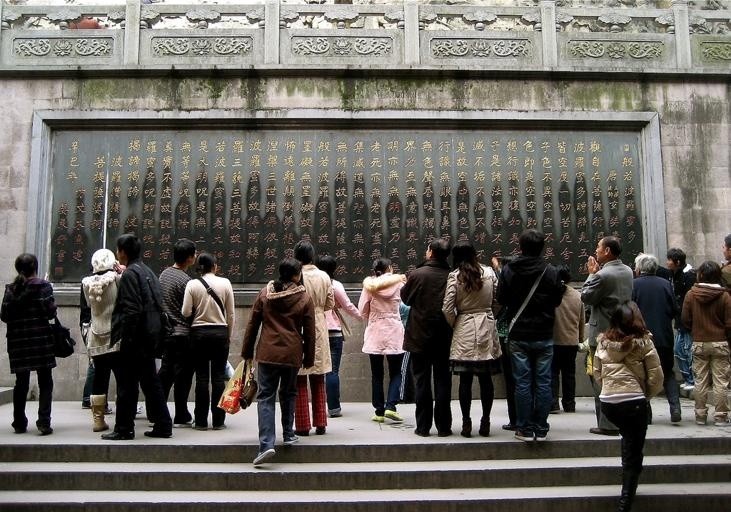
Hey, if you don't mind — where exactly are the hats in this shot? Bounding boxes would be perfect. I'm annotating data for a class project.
[91,247,117,273]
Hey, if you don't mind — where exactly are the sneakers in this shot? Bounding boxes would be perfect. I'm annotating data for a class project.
[696,419,708,425]
[173,419,195,428]
[104,406,113,415]
[438,429,453,437]
[414,427,431,437]
[293,430,310,436]
[316,426,326,435]
[384,410,404,422]
[372,415,384,423]
[714,419,731,426]
[536,431,548,441]
[253,449,275,465]
[192,419,208,430]
[671,414,682,424]
[16,428,27,433]
[213,424,228,430]
[39,426,54,434]
[283,435,300,445]
[514,429,534,441]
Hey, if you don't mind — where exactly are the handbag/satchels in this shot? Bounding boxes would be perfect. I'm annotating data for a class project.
[239,380,258,409]
[50,323,76,358]
[217,359,255,414]
[335,309,352,342]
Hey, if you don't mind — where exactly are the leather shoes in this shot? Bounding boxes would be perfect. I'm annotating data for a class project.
[590,427,620,436]
[501,422,515,430]
[144,429,173,439]
[101,430,136,439]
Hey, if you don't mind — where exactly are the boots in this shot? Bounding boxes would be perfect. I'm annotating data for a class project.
[90,395,109,432]
[460,417,473,437]
[479,417,491,437]
[616,437,646,512]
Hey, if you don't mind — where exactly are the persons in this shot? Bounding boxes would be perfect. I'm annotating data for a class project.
[589,297,666,512]
[79,266,97,409]
[81,248,124,432]
[180,251,237,431]
[293,240,336,436]
[159,237,198,428]
[357,256,408,423]
[0,252,58,435]
[241,255,317,466]
[316,255,366,418]
[98,233,173,440]
[401,226,730,442]
